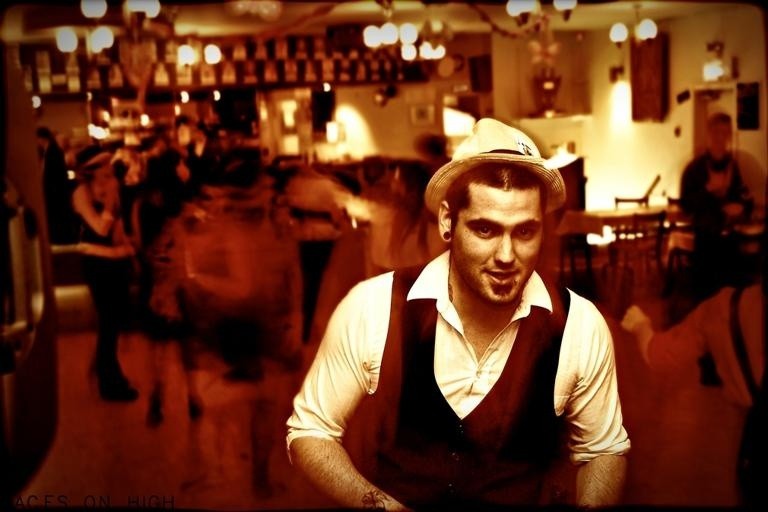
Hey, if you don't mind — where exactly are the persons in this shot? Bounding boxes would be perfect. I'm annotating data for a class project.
[36,112,768,511]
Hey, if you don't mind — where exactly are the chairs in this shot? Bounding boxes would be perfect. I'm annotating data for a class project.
[613,175,691,275]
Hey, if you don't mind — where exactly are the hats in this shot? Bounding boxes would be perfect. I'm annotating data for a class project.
[425,118,567,215]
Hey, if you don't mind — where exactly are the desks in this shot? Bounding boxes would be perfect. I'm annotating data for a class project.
[566,207,765,296]
